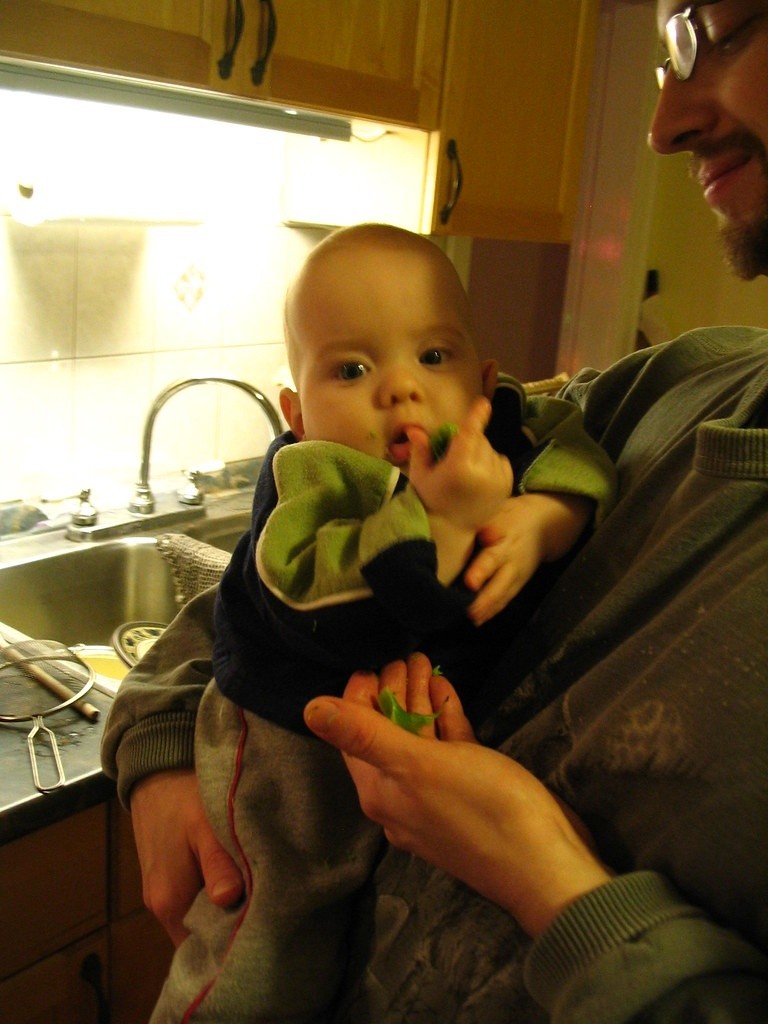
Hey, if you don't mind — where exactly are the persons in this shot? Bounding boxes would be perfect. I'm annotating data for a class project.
[150,223,620,1024]
[100,1,768,1024]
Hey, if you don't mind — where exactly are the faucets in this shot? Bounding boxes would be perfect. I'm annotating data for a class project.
[128,376,285,514]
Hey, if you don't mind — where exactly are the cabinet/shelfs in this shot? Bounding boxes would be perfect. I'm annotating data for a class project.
[0,0,451,142]
[283,0,616,247]
[0,764,174,1024]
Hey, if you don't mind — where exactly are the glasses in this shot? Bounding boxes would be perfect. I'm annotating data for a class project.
[656,0,714,93]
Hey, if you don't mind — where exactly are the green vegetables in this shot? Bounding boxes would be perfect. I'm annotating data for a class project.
[430,422,459,464]
[375,688,450,732]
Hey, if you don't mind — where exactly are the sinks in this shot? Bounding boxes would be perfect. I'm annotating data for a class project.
[171,509,252,555]
[0,531,230,649]
